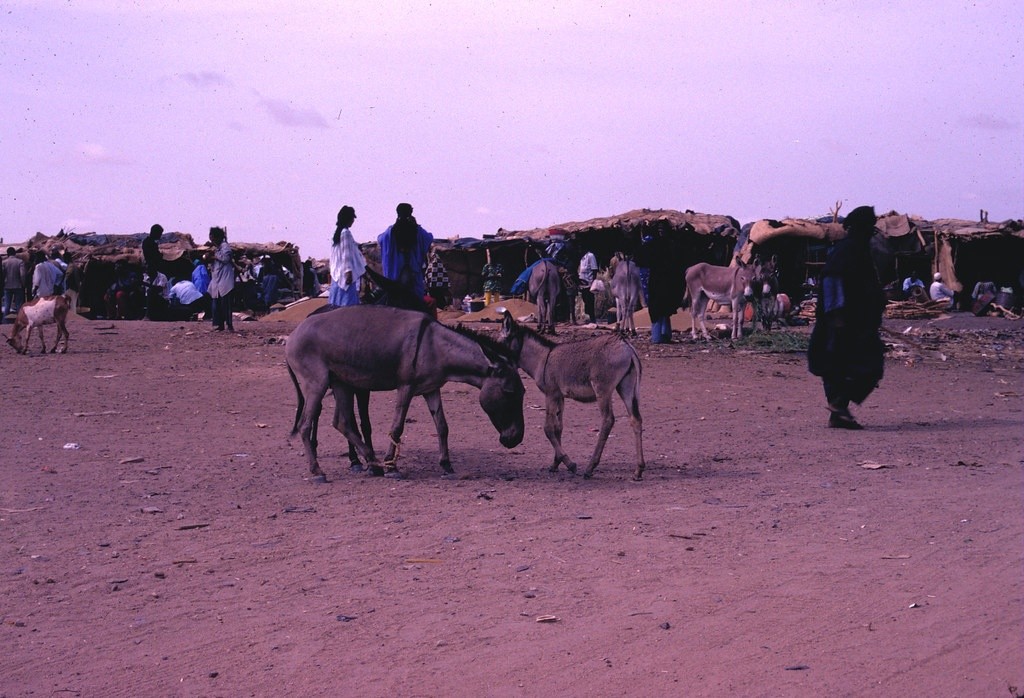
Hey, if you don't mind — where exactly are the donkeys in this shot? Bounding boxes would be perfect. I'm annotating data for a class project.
[609,256,643,337]
[284,264,527,484]
[499,309,647,480]
[1,294,71,355]
[527,261,561,335]
[682,253,781,343]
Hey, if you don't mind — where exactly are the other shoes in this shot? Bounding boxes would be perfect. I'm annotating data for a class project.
[934,273,941,280]
[829,409,863,429]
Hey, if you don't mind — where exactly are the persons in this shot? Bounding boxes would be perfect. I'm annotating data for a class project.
[142,224,165,320]
[902,269,959,313]
[329,206,368,309]
[807,206,887,429]
[377,203,434,304]
[207,228,236,333]
[481,229,678,345]
[0,246,323,325]
